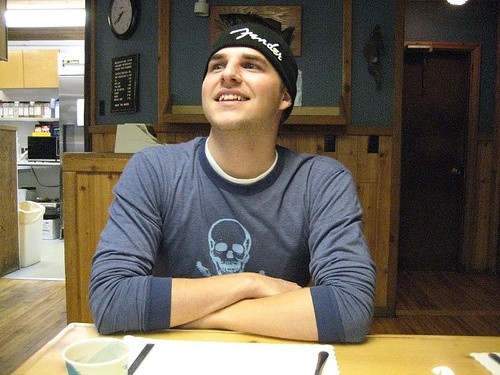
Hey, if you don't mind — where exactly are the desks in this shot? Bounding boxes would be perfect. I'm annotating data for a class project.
[11,320,500,375]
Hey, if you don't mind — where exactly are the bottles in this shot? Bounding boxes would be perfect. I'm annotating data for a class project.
[293,71,302,107]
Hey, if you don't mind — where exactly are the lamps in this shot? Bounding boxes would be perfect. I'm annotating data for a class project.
[194,0,209,17]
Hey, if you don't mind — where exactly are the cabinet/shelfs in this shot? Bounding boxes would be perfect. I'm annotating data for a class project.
[0,49,60,89]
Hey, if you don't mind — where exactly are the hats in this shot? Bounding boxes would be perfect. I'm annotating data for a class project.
[202,23,299,122]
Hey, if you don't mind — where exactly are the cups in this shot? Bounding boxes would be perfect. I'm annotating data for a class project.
[62,337,129,375]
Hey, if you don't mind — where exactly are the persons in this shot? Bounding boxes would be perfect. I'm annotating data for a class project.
[88,24,377,345]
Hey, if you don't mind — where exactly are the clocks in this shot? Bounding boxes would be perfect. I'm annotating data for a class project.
[107,0,141,41]
